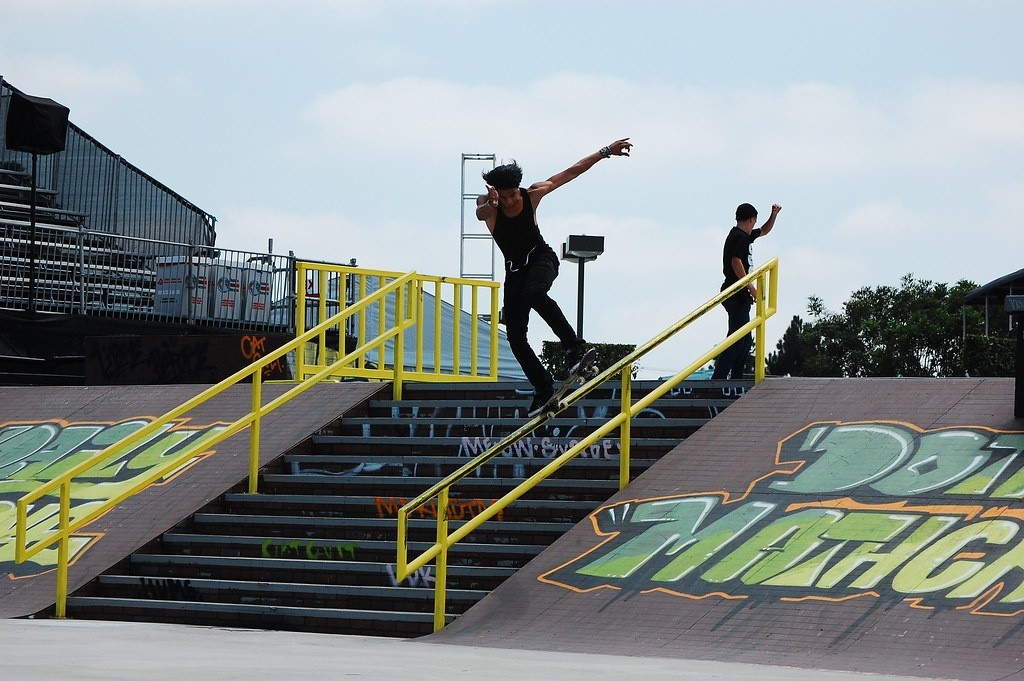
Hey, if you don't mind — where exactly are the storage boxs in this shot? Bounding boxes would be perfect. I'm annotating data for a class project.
[153,256,273,322]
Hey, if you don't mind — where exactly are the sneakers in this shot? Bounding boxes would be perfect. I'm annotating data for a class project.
[569,355,582,374]
[528,387,555,417]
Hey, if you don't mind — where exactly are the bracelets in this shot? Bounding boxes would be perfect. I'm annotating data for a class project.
[487,200,492,208]
[600,145,611,158]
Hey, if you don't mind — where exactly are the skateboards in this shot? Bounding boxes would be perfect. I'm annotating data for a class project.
[531,348,599,420]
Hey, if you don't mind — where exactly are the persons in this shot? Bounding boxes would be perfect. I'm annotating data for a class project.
[475,138,633,417]
[707,203,782,381]
